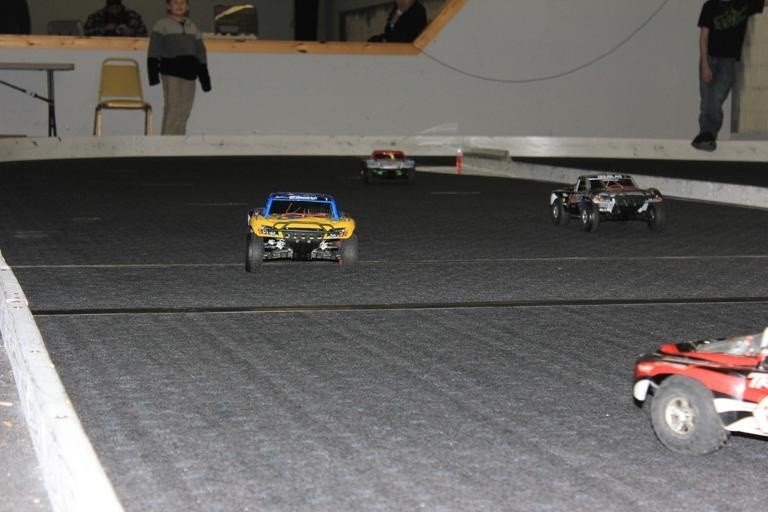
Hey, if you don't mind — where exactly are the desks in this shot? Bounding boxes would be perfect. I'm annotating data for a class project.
[0,61,76,137]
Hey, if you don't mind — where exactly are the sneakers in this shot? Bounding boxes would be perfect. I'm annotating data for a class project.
[691,132,717,152]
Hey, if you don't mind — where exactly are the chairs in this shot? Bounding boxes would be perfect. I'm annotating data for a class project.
[92,57,152,137]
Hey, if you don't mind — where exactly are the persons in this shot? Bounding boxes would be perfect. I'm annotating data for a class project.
[147,0,212,134]
[82,0,148,37]
[366,1,429,45]
[690,1,768,150]
